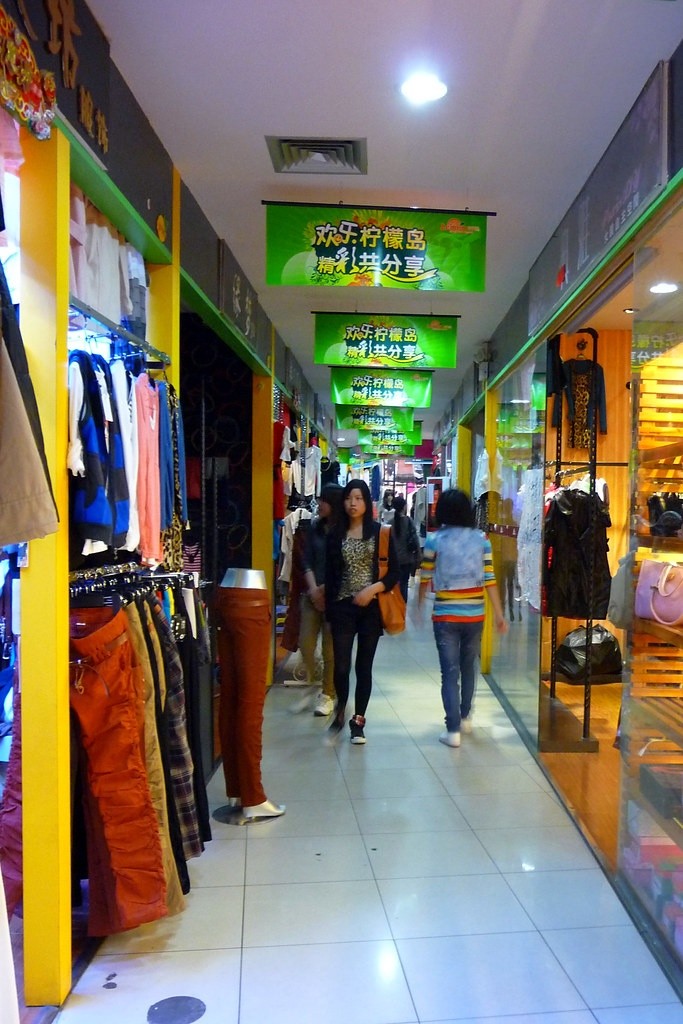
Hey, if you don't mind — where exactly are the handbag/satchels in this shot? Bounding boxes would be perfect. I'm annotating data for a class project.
[633,559,683,626]
[554,623,623,681]
[607,550,634,629]
[393,535,423,577]
[647,492,683,537]
[378,523,407,635]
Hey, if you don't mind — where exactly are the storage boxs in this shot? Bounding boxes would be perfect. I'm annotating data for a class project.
[623,848,683,957]
[640,835,683,872]
[639,763,683,820]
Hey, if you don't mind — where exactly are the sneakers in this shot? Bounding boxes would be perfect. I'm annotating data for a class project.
[289,687,323,713]
[313,693,337,717]
[439,731,461,748]
[322,715,346,745]
[349,714,366,743]
[461,719,472,733]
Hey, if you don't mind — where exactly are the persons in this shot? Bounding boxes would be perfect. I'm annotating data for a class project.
[418,489,505,748]
[499,498,517,622]
[323,479,400,744]
[214,567,285,819]
[280,478,423,718]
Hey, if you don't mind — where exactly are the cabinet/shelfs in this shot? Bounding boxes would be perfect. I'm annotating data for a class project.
[625,442,683,854]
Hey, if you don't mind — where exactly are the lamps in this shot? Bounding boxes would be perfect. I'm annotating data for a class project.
[473,348,489,381]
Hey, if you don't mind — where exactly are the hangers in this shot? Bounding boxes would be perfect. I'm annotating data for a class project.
[160,359,175,397]
[139,349,156,389]
[551,468,589,498]
[575,352,586,361]
[68,569,194,616]
